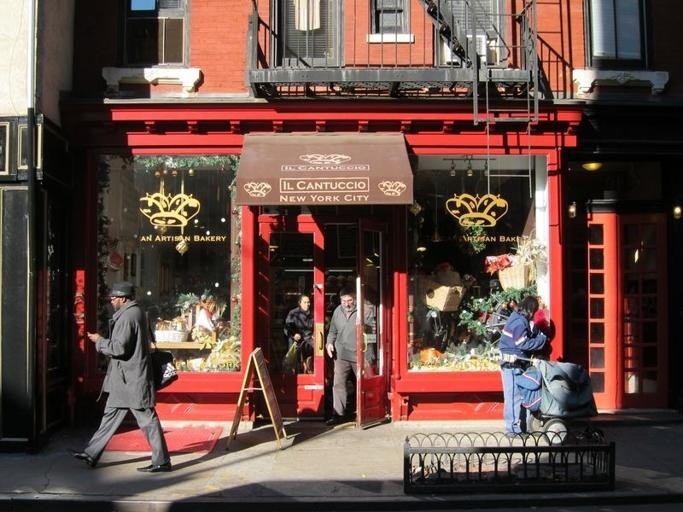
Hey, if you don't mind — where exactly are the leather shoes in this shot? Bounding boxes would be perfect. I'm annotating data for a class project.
[326,416,342,425]
[137,462,172,472]
[505,432,530,438]
[68,449,96,466]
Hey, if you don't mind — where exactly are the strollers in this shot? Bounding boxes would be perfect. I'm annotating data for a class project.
[514,355,598,447]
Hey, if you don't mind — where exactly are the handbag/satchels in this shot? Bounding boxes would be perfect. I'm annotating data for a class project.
[149,325,178,390]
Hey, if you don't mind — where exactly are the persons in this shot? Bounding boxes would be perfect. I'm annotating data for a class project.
[283,294,314,374]
[325,287,375,427]
[499,296,547,439]
[67,282,171,472]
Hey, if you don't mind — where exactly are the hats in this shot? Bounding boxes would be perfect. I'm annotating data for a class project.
[108,282,135,296]
